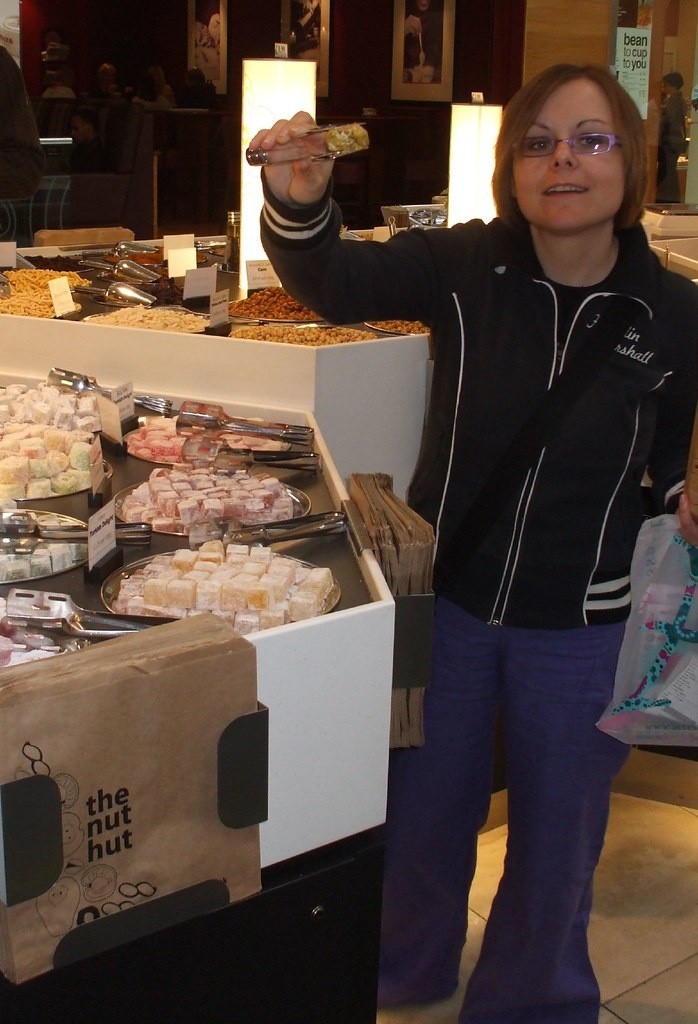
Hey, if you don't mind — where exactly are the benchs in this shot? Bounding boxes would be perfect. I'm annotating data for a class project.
[45,103,143,224]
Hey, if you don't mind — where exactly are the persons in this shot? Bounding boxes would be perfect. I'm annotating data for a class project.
[0,45,48,202]
[71,109,116,173]
[181,67,216,109]
[657,73,687,203]
[141,66,173,107]
[248,62,698,1024]
[89,63,138,101]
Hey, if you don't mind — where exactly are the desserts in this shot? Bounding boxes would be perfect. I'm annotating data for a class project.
[324,122,370,153]
[0,381,333,668]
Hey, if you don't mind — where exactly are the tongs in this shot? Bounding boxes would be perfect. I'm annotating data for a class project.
[1,512,154,547]
[47,368,173,415]
[183,431,320,463]
[187,510,347,545]
[246,120,369,166]
[4,587,181,641]
[177,401,314,447]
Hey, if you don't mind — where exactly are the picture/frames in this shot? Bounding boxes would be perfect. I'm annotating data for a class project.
[278,0,334,101]
[184,0,232,98]
[388,0,460,108]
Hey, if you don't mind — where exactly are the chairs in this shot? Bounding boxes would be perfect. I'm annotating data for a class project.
[332,148,374,224]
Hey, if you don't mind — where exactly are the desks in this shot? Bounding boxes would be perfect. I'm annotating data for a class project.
[156,107,239,224]
[318,115,424,226]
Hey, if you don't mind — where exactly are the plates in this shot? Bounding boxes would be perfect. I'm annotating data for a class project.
[0,508,88,584]
[114,480,312,536]
[80,312,214,336]
[365,320,417,335]
[228,300,326,323]
[8,460,114,502]
[101,550,341,630]
[54,302,83,319]
[122,426,290,466]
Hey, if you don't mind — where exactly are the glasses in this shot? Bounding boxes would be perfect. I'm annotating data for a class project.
[513,133,621,157]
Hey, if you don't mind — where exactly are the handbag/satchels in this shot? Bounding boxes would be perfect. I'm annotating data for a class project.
[596,513,698,747]
